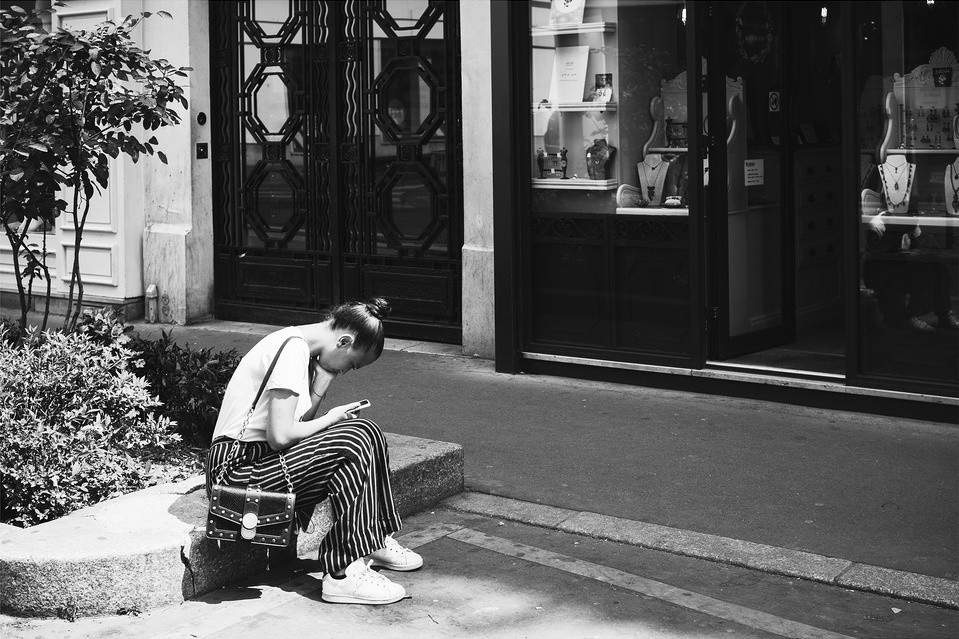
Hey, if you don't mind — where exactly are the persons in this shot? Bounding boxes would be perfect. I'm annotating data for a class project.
[203,296,424,606]
[875,226,959,333]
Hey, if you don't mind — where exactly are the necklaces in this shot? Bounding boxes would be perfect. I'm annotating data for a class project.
[943,160,958,217]
[876,159,913,214]
[641,160,667,201]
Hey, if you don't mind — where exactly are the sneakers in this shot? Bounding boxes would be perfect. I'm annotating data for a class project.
[364,535,423,571]
[321,557,405,605]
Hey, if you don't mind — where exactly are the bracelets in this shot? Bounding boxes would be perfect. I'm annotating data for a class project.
[311,386,327,399]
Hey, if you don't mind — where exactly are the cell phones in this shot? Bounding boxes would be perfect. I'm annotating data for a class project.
[344,398,371,414]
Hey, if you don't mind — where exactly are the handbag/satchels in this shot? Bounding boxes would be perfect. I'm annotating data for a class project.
[206,484,298,548]
